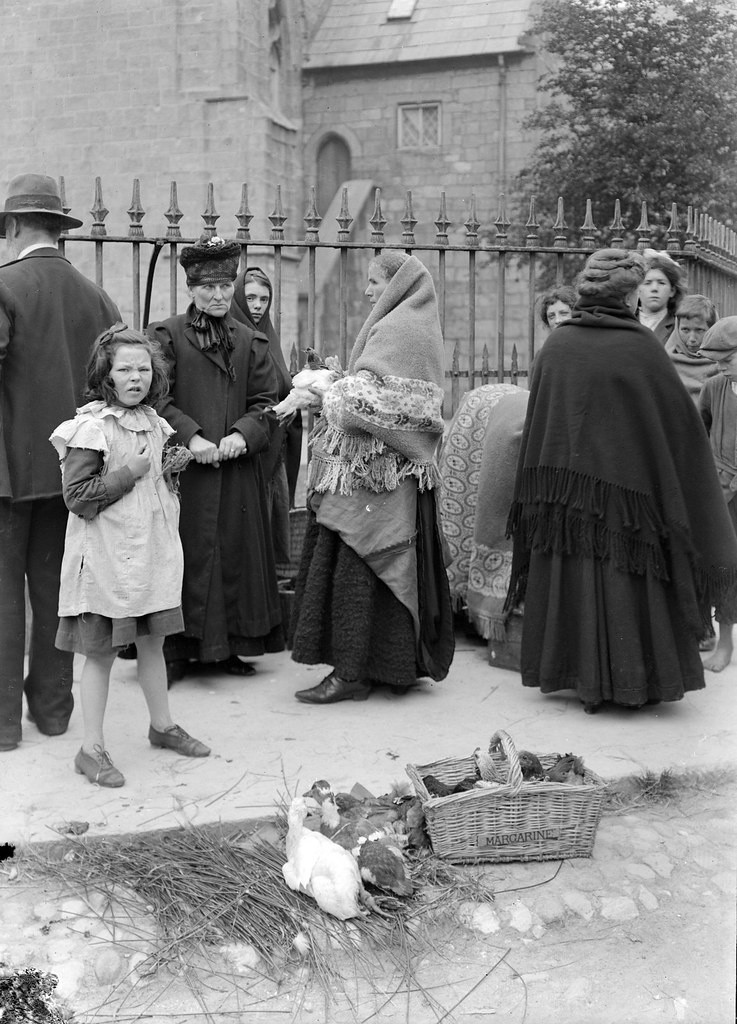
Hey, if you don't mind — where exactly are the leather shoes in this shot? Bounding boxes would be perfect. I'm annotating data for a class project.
[296,672,371,702]
[395,683,408,694]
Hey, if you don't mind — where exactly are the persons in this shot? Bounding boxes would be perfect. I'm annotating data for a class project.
[697,315,737,673]
[502,248,737,717]
[229,266,303,512]
[0,175,121,752]
[145,235,292,691]
[531,285,579,333]
[290,249,456,705]
[49,322,211,788]
[634,249,690,350]
[663,294,720,415]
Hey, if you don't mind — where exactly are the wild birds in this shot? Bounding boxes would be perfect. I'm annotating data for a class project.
[281,797,412,921]
[302,781,426,922]
[260,346,346,428]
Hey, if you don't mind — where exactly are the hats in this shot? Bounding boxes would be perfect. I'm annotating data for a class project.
[179,235,242,286]
[696,316,737,360]
[0,174,84,236]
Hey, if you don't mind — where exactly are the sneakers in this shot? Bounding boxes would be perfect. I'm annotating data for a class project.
[75,744,127,787]
[149,723,211,758]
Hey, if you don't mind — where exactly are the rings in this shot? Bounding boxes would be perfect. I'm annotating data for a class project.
[230,449,235,453]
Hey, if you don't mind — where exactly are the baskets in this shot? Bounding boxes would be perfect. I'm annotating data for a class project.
[408,731,608,863]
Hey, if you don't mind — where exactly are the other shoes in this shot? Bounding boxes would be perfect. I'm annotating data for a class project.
[224,654,255,674]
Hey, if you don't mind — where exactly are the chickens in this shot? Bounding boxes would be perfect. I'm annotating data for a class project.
[423,746,588,798]
